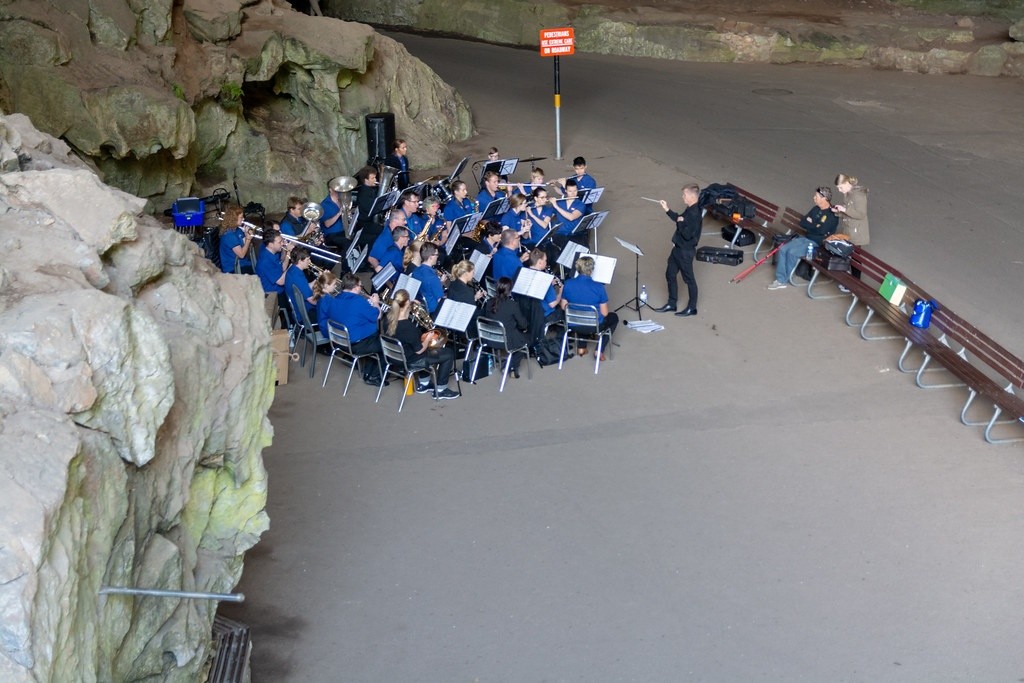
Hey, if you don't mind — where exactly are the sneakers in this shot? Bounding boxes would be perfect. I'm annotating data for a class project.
[432,388,460,399]
[416,380,434,393]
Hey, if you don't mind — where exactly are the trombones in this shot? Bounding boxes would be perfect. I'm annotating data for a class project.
[240,221,390,313]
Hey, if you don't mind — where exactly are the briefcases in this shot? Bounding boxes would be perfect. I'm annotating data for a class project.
[814,246,850,271]
[463,354,489,382]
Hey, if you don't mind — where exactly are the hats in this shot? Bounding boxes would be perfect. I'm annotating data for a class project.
[817,186,833,207]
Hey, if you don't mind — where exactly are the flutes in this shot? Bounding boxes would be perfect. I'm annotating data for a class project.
[546,196,580,201]
[499,183,547,187]
[526,208,528,220]
[489,238,501,256]
[550,173,587,184]
[472,278,486,298]
[436,261,450,284]
[546,264,561,287]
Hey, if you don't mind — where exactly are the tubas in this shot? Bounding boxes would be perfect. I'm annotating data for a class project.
[373,165,400,227]
[329,176,359,240]
[301,201,325,246]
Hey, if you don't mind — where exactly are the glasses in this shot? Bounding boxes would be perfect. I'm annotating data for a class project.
[431,253,440,258]
[401,235,409,239]
[408,201,420,204]
[537,196,548,199]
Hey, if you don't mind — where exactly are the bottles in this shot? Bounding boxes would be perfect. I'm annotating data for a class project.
[404,374,413,395]
[806,243,813,261]
[288,324,295,348]
[487,353,493,375]
[640,285,648,305]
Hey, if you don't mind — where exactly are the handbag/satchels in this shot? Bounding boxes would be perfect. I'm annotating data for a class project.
[823,233,853,258]
[534,332,570,369]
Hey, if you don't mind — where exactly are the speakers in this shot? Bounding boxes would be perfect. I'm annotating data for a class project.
[365,112,395,161]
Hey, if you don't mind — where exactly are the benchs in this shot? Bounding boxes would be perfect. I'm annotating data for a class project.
[701,182,1024,444]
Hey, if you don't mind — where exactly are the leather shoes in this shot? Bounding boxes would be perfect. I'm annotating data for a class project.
[364,373,369,379]
[675,307,697,316]
[655,305,677,312]
[366,377,390,387]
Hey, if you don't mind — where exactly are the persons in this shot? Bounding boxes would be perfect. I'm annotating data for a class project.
[528,248,575,361]
[421,195,447,250]
[766,186,839,290]
[831,173,870,292]
[255,197,331,336]
[328,272,390,386]
[385,138,409,189]
[550,157,596,240]
[380,288,460,400]
[559,257,619,361]
[519,187,555,246]
[480,147,507,191]
[474,171,505,214]
[369,190,530,378]
[313,273,340,355]
[358,166,384,233]
[550,178,590,252]
[444,180,479,249]
[506,167,545,202]
[482,221,503,255]
[219,206,254,274]
[319,177,352,279]
[492,229,529,281]
[501,193,533,267]
[655,181,703,317]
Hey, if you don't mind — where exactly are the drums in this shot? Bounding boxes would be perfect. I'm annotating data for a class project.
[432,177,453,204]
[410,182,432,209]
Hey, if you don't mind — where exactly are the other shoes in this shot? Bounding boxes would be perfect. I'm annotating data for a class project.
[838,284,850,292]
[500,363,521,379]
[343,355,358,370]
[768,280,786,290]
[316,345,336,357]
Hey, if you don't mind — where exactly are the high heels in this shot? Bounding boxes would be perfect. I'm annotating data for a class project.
[594,351,606,361]
[578,348,588,356]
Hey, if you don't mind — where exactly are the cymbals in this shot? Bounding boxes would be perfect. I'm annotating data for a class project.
[517,157,546,162]
[427,174,450,180]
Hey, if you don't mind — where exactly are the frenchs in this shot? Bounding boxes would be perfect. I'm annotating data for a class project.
[411,302,448,347]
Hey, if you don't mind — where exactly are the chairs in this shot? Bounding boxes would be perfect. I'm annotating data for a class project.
[202,160,613,413]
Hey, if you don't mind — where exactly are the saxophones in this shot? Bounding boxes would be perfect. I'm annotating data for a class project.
[404,186,507,243]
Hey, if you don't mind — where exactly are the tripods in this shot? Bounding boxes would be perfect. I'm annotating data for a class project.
[614,244,655,321]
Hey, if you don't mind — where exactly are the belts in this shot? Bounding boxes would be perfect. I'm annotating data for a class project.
[351,338,364,348]
